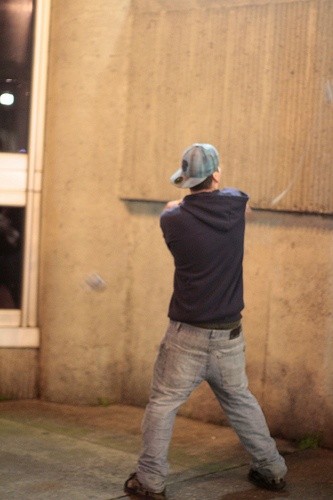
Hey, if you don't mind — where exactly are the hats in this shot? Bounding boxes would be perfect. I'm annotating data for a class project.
[169,143,219,190]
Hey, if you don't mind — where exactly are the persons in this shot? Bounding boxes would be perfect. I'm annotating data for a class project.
[124,144,287,500]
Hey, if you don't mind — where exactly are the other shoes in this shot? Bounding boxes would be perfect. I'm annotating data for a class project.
[248,468,287,490]
[124,471,168,500]
